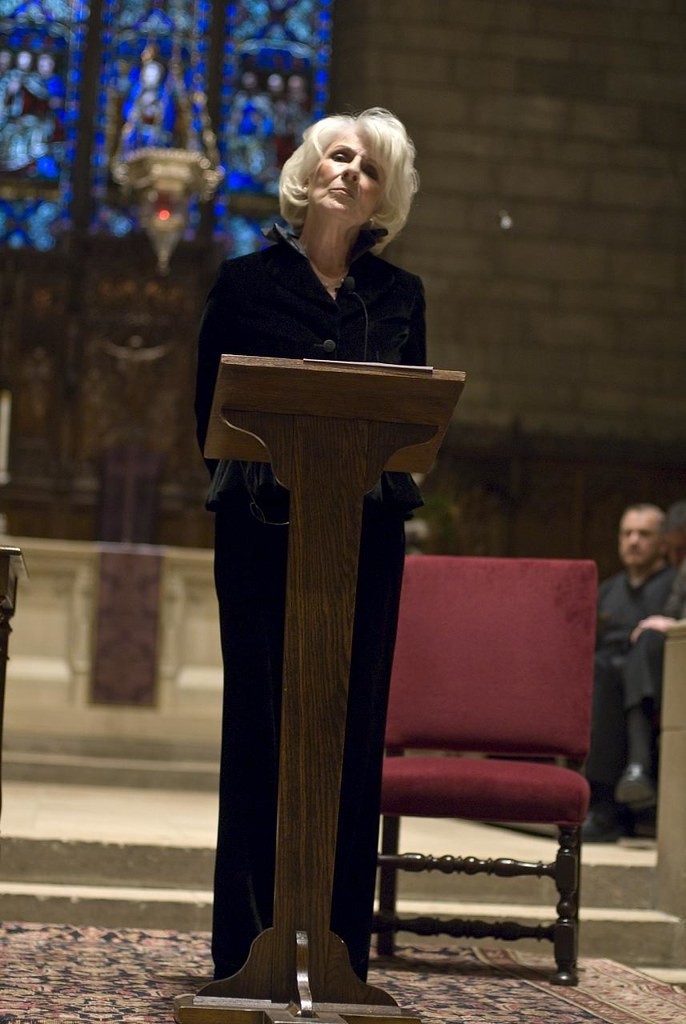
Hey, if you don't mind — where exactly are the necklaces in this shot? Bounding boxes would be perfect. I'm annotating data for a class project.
[320,271,349,287]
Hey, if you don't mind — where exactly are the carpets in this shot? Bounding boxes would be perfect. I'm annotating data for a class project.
[0,919,686,1024]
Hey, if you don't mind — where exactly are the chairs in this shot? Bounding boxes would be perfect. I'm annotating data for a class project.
[379,554,598,985]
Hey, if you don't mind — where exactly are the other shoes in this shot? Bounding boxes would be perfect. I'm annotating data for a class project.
[614,770,656,811]
[581,799,616,842]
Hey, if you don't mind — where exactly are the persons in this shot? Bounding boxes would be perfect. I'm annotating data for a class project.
[580,501,686,843]
[195,108,428,984]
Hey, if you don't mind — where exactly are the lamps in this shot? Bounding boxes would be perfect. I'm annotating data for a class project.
[111,0,226,277]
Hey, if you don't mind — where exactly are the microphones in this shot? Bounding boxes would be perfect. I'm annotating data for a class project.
[344,276,369,361]
